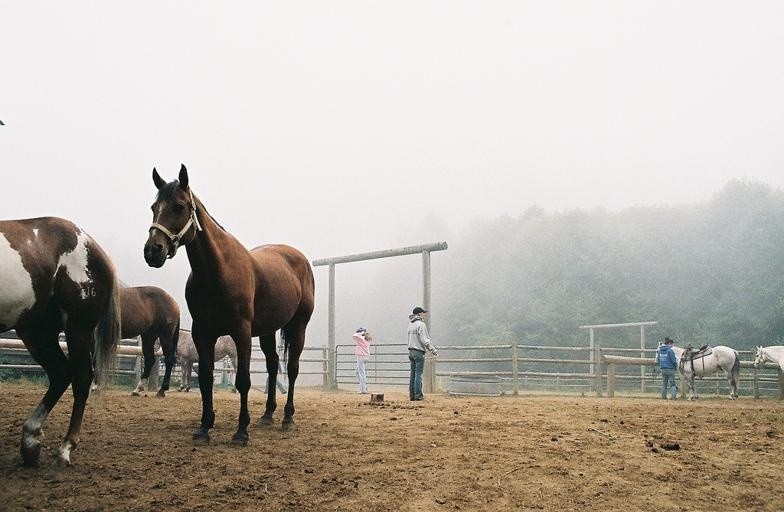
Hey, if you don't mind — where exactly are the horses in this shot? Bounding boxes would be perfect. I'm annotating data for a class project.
[142,163,315,445]
[754,345,784,371]
[90,285,180,399]
[154,328,238,393]
[654,341,740,402]
[0,216,123,471]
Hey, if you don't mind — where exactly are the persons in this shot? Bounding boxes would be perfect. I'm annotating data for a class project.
[658,336,678,400]
[407,306,438,401]
[352,327,374,394]
[264,362,288,396]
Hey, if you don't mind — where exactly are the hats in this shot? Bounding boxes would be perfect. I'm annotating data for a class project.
[414,307,427,315]
